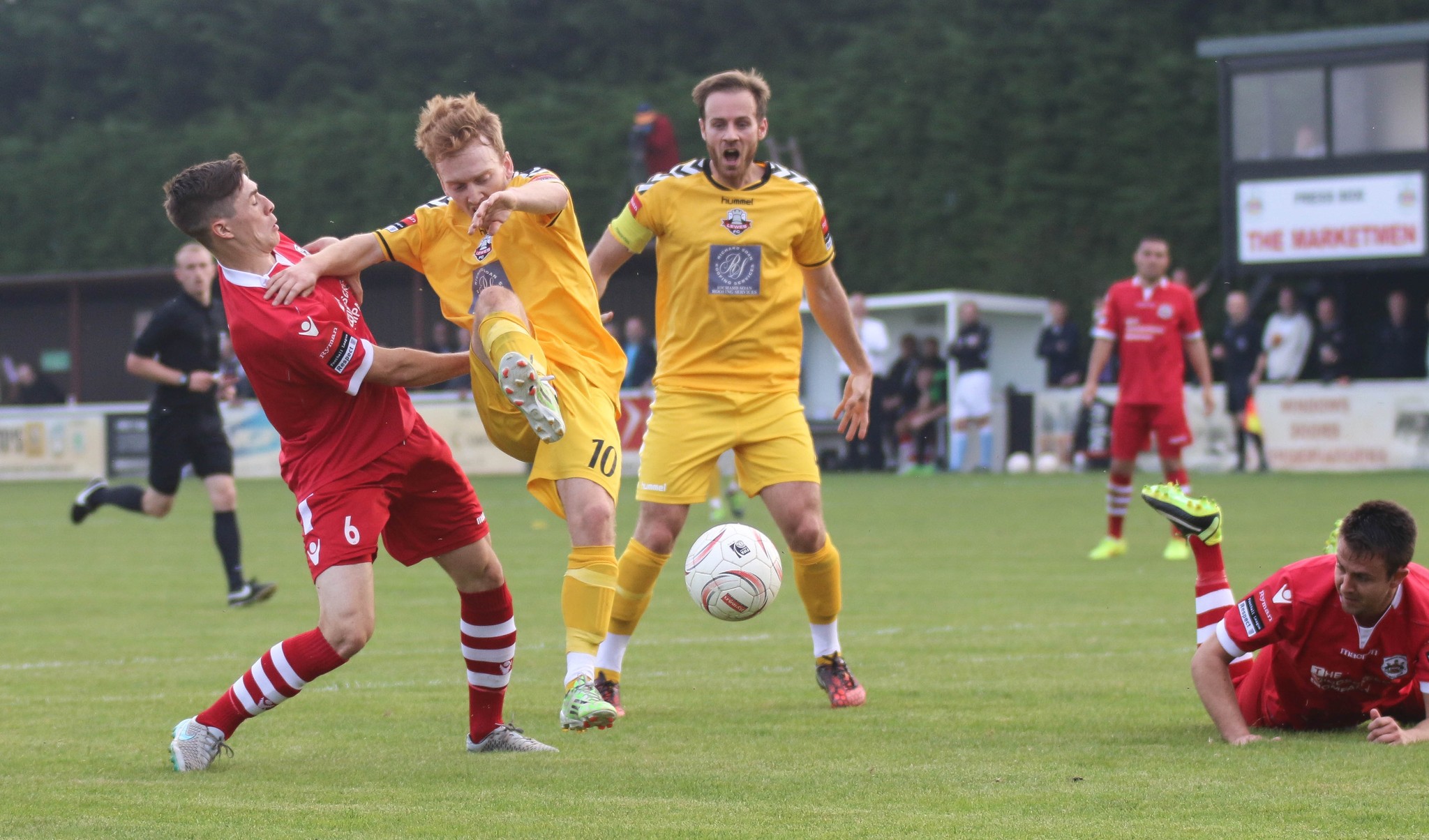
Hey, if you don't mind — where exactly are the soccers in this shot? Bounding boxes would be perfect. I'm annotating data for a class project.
[685,523,783,622]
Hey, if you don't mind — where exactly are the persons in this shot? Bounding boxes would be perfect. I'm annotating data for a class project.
[383,286,954,472]
[66,243,277,610]
[580,69,875,709]
[1076,224,1216,560]
[1205,287,1276,473]
[1134,473,1429,744]
[1280,123,1329,158]
[1084,261,1261,386]
[1305,296,1359,383]
[158,153,558,778]
[1363,289,1427,378]
[260,92,623,734]
[946,301,996,475]
[6,313,255,421]
[1030,295,1082,385]
[1255,286,1315,382]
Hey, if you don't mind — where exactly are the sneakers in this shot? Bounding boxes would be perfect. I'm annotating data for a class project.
[167,714,227,772]
[560,676,618,733]
[1142,481,1223,547]
[816,652,866,710]
[594,677,625,721]
[467,725,560,754]
[1322,517,1344,555]
[72,476,110,523]
[228,583,277,608]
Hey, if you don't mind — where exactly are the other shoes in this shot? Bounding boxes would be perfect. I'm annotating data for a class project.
[1163,540,1189,560]
[1088,537,1129,560]
